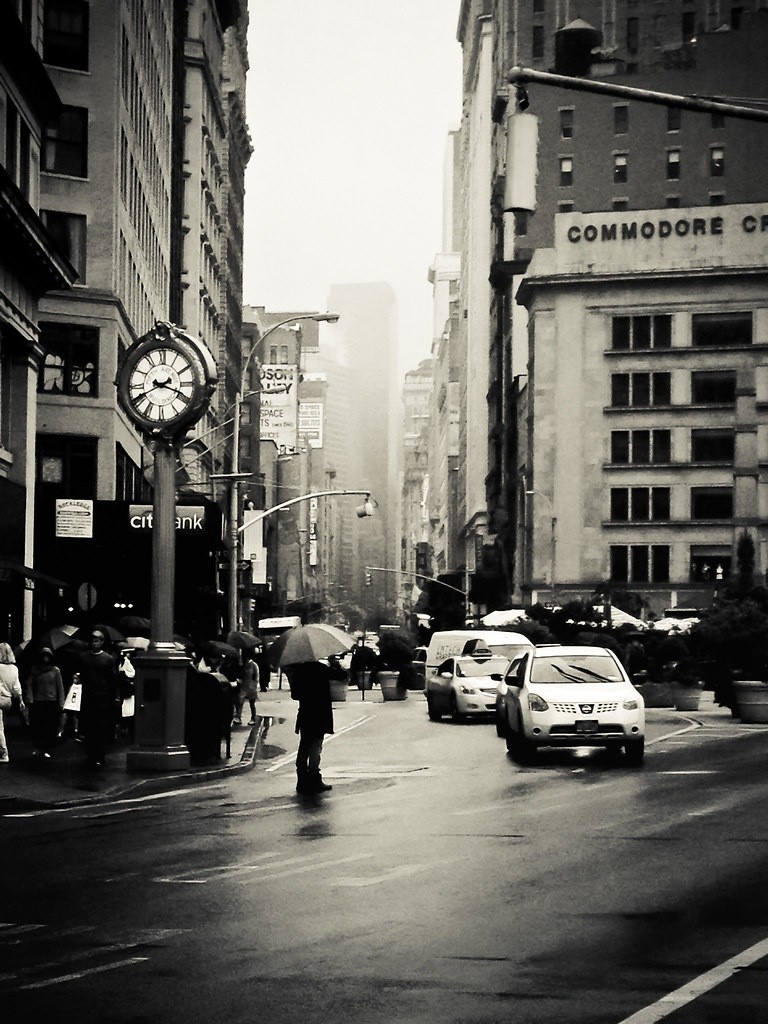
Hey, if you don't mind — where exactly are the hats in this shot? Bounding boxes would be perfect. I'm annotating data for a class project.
[90,631,105,639]
[41,647,53,656]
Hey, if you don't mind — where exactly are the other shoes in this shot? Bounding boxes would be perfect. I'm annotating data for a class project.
[0,756,9,763]
[44,752,51,758]
[234,718,241,724]
[248,720,256,724]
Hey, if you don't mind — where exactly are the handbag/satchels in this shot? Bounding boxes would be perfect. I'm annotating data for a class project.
[63,683,83,712]
[123,695,135,717]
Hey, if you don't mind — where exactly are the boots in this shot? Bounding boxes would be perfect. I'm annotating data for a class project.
[296,768,332,795]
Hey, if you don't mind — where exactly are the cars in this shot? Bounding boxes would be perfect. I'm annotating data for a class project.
[426,639,516,722]
[492,644,644,765]
[412,646,428,690]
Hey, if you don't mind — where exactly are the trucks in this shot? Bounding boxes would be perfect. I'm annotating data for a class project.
[257,616,302,638]
[425,630,536,680]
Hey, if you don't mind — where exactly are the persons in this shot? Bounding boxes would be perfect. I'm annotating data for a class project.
[283,654,348,795]
[72,639,120,764]
[26,647,65,759]
[0,642,26,762]
[220,649,261,725]
[260,646,270,693]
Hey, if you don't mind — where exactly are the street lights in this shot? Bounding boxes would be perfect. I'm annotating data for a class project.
[230,310,340,632]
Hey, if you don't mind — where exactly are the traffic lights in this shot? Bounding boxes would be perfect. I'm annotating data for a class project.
[365,573,371,586]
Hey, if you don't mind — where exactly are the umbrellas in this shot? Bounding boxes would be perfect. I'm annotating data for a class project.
[198,640,238,659]
[70,625,130,643]
[23,625,79,651]
[268,622,357,667]
[224,631,263,649]
[115,616,151,627]
